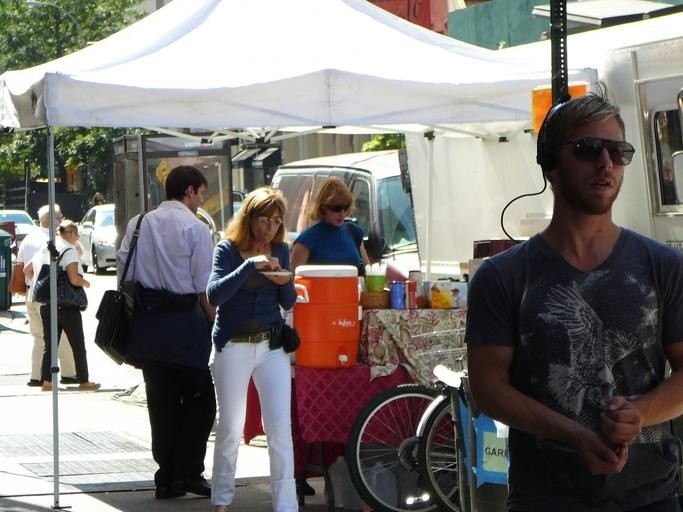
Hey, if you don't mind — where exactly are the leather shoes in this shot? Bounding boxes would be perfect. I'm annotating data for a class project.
[155,485,186,500]
[183,475,211,496]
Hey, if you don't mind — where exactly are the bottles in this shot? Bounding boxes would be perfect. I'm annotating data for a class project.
[391,279,405,310]
[405,280,418,309]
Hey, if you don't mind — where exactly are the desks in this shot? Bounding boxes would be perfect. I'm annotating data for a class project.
[287,363,456,512]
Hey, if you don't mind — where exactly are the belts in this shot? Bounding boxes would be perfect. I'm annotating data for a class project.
[230,331,270,344]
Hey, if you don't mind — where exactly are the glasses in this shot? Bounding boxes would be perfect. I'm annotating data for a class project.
[328,204,350,212]
[257,216,283,224]
[570,136,636,166]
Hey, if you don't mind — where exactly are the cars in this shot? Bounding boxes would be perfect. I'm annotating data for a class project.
[0,209,40,247]
[79,203,116,275]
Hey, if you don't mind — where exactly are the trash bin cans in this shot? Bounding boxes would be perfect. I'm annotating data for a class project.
[0,229,14,311]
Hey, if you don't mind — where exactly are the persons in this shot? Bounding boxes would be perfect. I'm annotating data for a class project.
[92,192,105,205]
[289,176,370,496]
[466,94,683,512]
[116,166,216,501]
[23,219,100,390]
[207,185,301,512]
[16,202,81,386]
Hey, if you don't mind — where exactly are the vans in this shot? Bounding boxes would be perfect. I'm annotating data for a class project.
[271,149,422,272]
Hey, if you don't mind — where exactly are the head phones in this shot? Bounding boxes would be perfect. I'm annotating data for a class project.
[536,95,574,171]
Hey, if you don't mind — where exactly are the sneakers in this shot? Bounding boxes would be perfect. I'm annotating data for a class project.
[42,383,52,391]
[78,383,101,390]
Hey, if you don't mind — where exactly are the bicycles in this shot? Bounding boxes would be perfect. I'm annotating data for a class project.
[346,389,462,512]
[417,362,508,512]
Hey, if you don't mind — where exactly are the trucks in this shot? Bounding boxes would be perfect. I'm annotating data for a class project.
[406,11,683,277]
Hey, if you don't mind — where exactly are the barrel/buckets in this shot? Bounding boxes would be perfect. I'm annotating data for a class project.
[292,266,359,367]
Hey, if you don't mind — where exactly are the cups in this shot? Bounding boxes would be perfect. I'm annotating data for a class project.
[366,274,386,292]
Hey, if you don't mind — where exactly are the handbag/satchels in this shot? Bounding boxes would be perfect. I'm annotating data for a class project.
[32,248,87,312]
[93,212,144,366]
[8,263,26,293]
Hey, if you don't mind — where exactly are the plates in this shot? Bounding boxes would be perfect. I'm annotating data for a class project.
[258,271,292,276]
